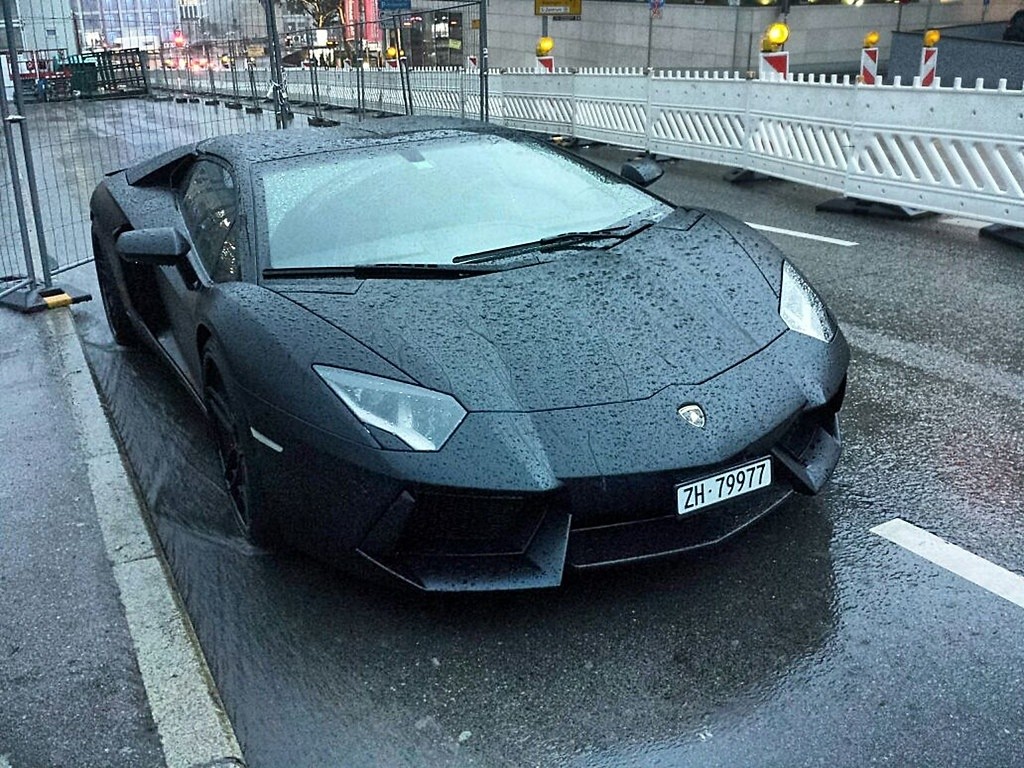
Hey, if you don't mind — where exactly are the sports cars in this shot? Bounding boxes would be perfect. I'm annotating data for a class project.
[87,116,852,607]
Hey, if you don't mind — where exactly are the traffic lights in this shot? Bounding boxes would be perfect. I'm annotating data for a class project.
[175,31,182,46]
